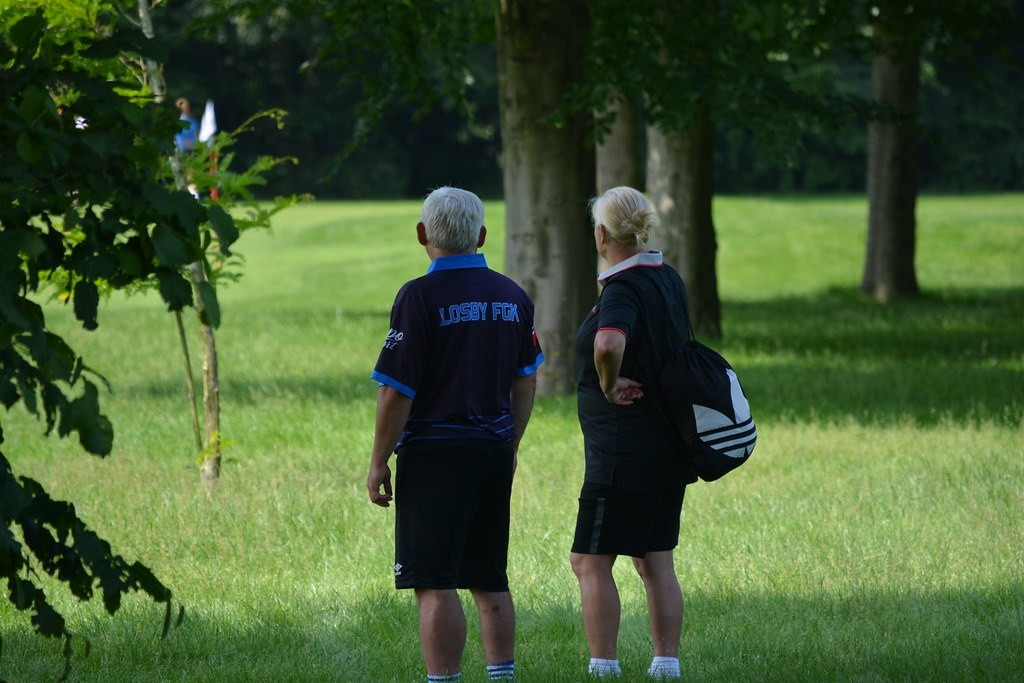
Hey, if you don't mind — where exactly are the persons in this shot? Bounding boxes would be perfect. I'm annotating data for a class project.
[176,98,199,204]
[366,185,546,683]
[569,185,699,681]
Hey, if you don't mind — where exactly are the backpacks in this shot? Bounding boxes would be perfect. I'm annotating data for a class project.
[622,265,758,481]
